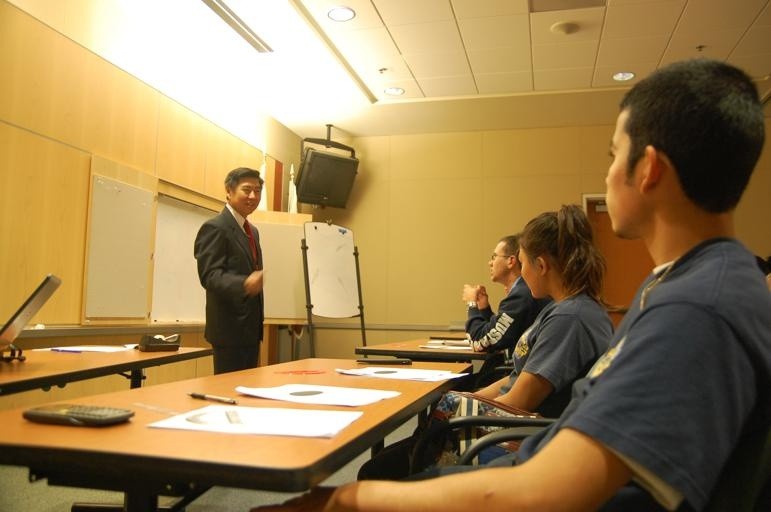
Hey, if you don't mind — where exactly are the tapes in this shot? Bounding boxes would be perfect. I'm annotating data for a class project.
[154,334,178,342]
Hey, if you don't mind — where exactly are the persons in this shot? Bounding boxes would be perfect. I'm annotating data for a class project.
[193,167,264,375]
[357,204,617,481]
[430,235,554,411]
[503,284,508,296]
[249,61,771,512]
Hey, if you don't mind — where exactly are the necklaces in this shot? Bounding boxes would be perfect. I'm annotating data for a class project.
[465,301,478,310]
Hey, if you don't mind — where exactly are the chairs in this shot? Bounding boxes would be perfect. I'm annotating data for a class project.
[409,354,556,477]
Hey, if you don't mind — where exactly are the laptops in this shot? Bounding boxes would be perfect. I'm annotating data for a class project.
[1,272,64,348]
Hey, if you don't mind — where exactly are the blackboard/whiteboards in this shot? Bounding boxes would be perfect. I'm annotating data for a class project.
[304,222,361,319]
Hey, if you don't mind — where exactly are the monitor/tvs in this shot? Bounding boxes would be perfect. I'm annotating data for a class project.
[296,125,360,210]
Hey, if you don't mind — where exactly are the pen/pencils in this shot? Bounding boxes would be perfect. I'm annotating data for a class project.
[187,393,235,404]
[52,348,82,353]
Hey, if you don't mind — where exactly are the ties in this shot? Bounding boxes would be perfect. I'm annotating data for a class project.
[244,221,258,261]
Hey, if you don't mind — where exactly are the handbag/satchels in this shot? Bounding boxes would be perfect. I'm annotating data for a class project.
[408,387,557,475]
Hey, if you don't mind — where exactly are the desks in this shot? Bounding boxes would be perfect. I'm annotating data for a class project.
[0,359,474,512]
[355,339,504,363]
[430,331,467,338]
[0,344,214,396]
